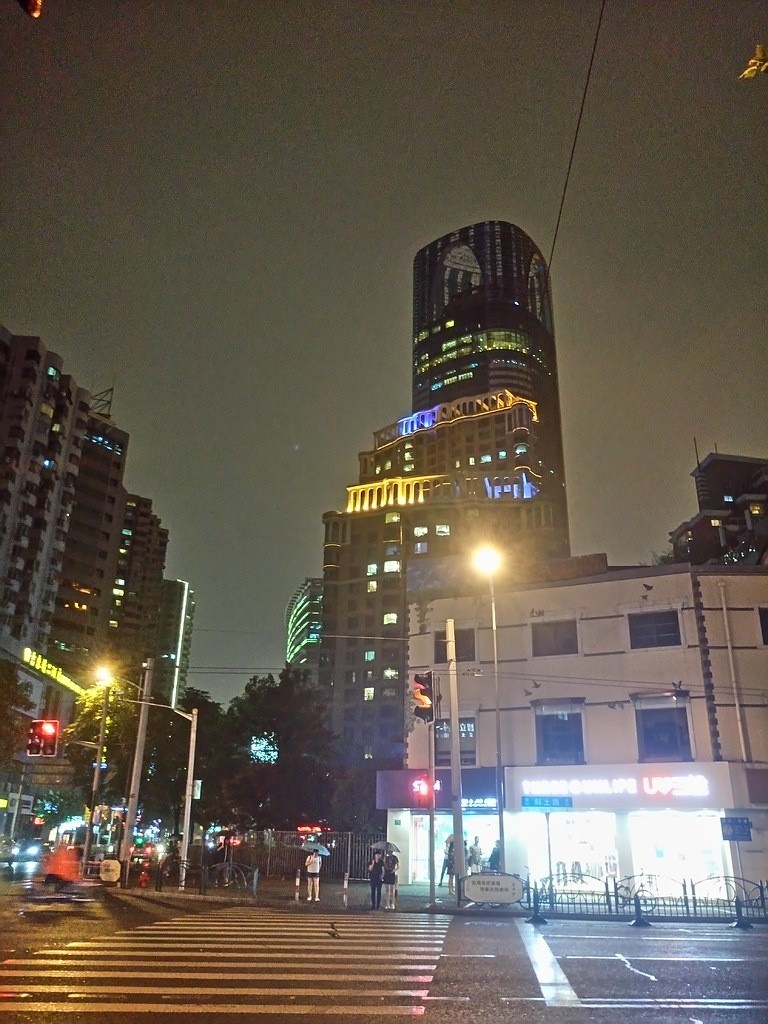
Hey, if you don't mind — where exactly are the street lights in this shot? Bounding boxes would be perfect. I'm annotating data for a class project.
[466,542,505,874]
[96,666,154,887]
[79,674,116,879]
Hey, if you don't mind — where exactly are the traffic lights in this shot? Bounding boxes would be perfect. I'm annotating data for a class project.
[41,719,60,758]
[412,774,430,808]
[25,719,45,756]
[412,670,437,726]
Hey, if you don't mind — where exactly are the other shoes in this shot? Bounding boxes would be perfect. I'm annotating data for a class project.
[449,890,455,895]
[371,905,376,910]
[385,904,395,910]
[307,897,312,901]
[376,906,380,910]
[314,898,320,902]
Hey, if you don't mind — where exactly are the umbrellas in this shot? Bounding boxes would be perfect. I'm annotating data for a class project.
[136,842,156,847]
[217,830,237,837]
[370,841,401,853]
[167,833,183,840]
[301,842,330,860]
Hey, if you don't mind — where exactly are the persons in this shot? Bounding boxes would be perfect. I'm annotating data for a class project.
[368,851,400,909]
[213,837,232,886]
[438,836,485,896]
[132,846,158,878]
[166,842,179,877]
[305,849,322,901]
[487,840,500,872]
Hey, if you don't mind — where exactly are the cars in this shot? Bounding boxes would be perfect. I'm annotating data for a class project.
[14,839,44,860]
[0,837,21,866]
